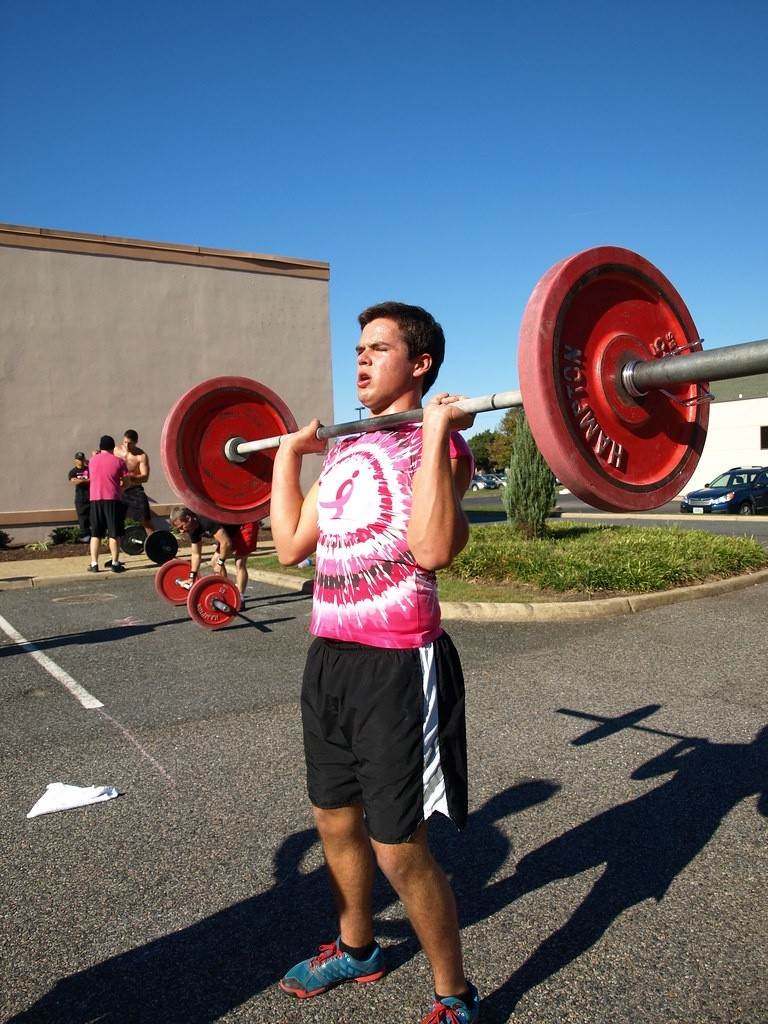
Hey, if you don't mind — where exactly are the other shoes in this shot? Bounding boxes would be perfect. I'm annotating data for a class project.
[112,562,125,572]
[87,564,98,572]
[239,600,245,612]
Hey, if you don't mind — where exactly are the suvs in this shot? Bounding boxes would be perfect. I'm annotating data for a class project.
[681,466,768,517]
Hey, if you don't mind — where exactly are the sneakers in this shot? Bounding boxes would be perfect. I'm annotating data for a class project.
[421,984,479,1024]
[279,936,387,999]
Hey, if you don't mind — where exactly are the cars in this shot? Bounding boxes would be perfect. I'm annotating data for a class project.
[467,474,498,492]
[482,474,508,490]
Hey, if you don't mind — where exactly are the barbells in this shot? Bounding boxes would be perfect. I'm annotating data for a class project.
[120,524,178,564]
[161,245,768,527]
[155,559,243,629]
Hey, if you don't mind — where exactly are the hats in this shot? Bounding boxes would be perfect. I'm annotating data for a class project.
[75,452,84,459]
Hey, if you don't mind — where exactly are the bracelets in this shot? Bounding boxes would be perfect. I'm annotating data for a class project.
[189,571,198,579]
[215,558,225,566]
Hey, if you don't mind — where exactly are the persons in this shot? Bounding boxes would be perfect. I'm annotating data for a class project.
[67,430,258,612]
[270,300,480,1024]
